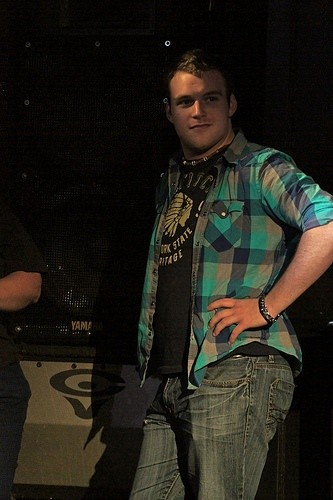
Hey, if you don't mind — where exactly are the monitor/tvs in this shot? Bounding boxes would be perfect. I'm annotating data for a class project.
[56,0,164,33]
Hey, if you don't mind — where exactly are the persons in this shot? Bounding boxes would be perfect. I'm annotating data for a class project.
[129,49,332,500]
[0,171,44,500]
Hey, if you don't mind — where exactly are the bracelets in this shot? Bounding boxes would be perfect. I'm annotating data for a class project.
[259,296,280,324]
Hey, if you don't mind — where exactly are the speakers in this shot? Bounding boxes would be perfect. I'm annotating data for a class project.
[22,34,193,357]
[264,396,333,500]
